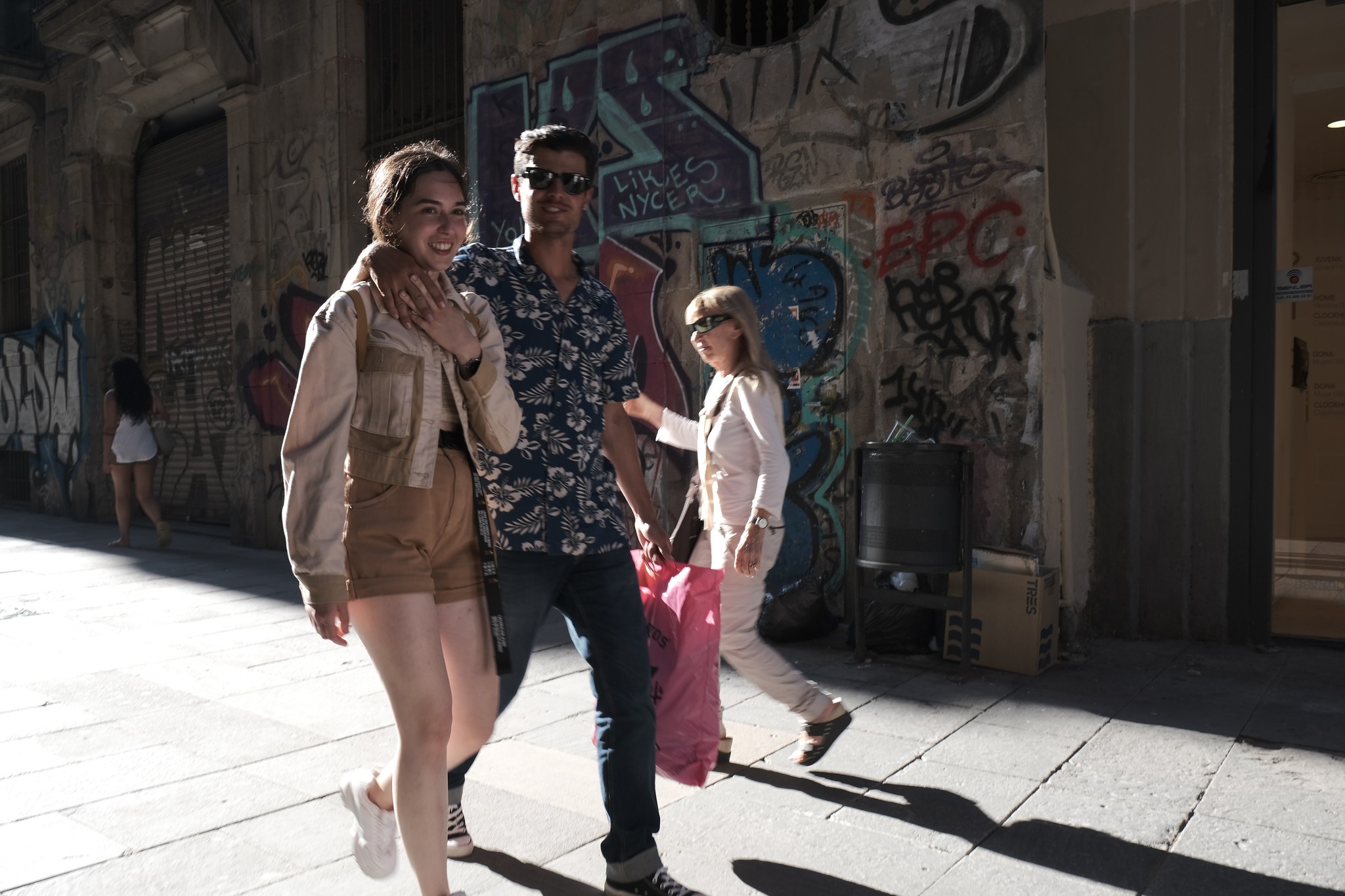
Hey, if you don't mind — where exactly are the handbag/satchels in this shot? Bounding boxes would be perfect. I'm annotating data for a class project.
[629,549,726,789]
[150,396,177,455]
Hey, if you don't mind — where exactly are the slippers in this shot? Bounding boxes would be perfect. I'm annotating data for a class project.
[107,539,130,548]
[157,520,173,549]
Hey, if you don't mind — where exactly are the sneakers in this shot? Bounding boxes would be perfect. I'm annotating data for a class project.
[605,865,705,896]
[339,766,399,880]
[444,800,474,860]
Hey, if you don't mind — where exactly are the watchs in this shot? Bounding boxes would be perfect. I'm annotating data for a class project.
[458,358,481,378]
[748,516,769,529]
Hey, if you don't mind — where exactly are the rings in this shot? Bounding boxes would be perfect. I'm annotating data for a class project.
[749,562,757,568]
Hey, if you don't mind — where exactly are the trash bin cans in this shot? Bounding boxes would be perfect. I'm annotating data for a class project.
[854,439,973,573]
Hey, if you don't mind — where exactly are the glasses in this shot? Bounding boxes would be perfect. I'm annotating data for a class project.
[685,313,734,338]
[517,166,594,196]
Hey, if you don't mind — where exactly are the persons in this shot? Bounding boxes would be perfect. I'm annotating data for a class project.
[620,286,852,770]
[100,357,177,550]
[340,125,706,896]
[281,142,521,896]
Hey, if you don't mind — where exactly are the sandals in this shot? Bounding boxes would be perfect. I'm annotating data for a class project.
[716,738,733,765]
[797,710,852,767]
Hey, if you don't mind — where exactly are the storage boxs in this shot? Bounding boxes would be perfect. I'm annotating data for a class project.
[944,567,1060,675]
